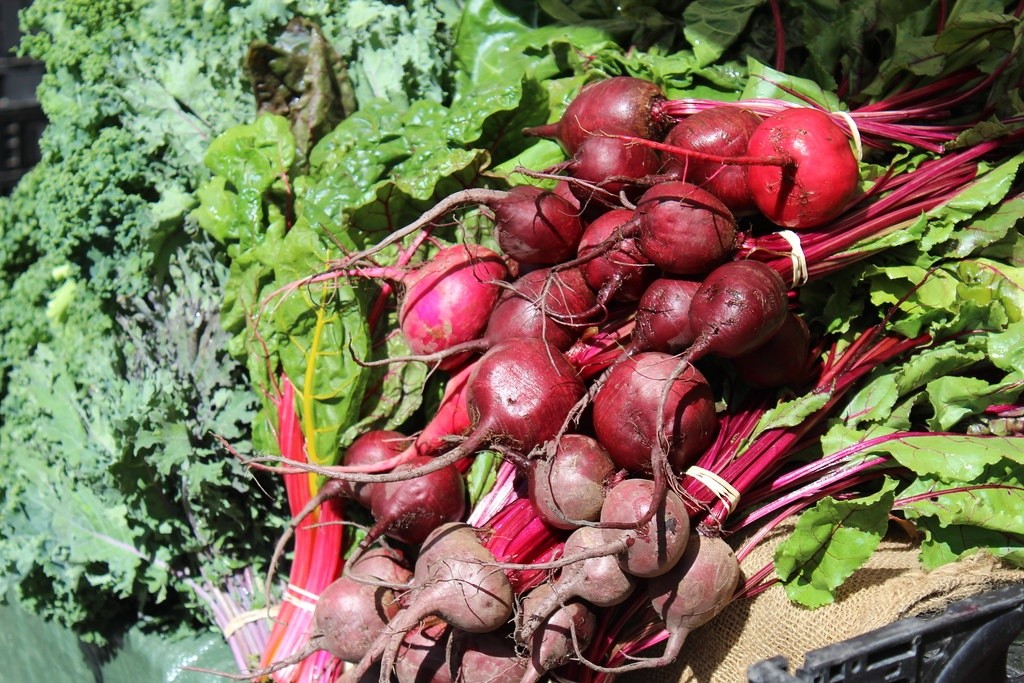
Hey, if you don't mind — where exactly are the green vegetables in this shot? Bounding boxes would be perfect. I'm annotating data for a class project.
[0,0,473,683]
